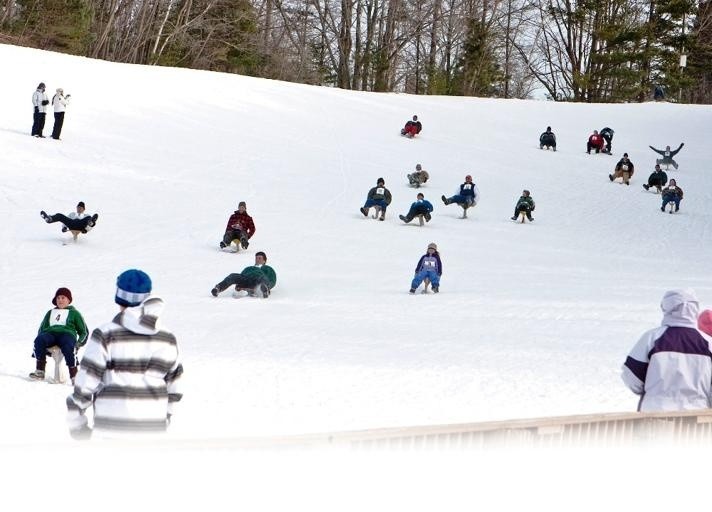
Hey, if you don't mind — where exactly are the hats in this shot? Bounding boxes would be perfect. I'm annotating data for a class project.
[660,287,699,321]
[115,269,152,306]
[52,288,73,307]
[238,201,247,210]
[77,202,86,210]
[696,308,712,338]
[377,178,385,184]
[427,243,437,250]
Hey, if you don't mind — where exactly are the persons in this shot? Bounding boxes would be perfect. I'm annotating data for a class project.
[218,201,255,250]
[39,200,99,233]
[400,114,421,138]
[27,287,88,387]
[587,126,686,213]
[510,190,536,222]
[406,163,430,188]
[66,266,185,441]
[211,252,277,299]
[539,126,557,152]
[408,240,442,293]
[441,175,478,208]
[358,177,392,221]
[31,82,49,138]
[619,287,712,411]
[50,87,72,140]
[399,192,434,224]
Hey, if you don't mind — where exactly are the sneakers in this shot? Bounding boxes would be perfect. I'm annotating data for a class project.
[240,237,249,249]
[441,195,449,205]
[432,286,438,292]
[260,283,270,298]
[379,213,386,221]
[410,288,416,292]
[425,212,431,221]
[511,215,518,220]
[211,288,218,297]
[88,213,98,227]
[29,370,45,380]
[360,207,369,216]
[399,214,408,222]
[529,218,535,222]
[40,210,51,222]
[220,241,226,249]
